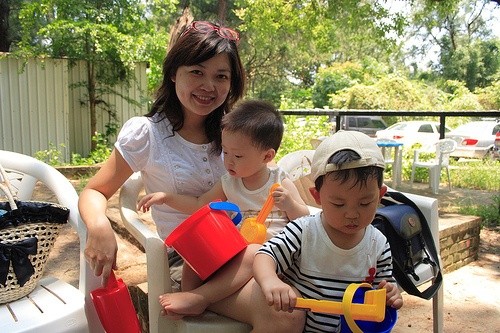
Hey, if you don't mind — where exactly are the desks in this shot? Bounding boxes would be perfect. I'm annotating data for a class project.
[376,141,404,190]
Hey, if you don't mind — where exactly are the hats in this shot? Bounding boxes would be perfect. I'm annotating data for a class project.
[311,130,386,183]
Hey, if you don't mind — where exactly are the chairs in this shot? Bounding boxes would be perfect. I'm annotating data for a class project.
[0,149,104,333]
[410,138,458,195]
[309,136,403,189]
[117,171,254,333]
[277,148,444,333]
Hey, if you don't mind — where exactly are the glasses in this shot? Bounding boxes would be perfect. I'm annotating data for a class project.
[179,22,240,48]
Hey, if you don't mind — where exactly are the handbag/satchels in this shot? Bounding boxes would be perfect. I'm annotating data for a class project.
[0,165,69,303]
[372,204,436,281]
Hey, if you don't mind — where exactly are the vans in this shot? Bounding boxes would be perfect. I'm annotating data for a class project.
[329,114,388,138]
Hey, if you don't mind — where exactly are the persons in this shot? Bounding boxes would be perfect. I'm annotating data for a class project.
[135,99,309,322]
[251,131,403,333]
[77,19,305,333]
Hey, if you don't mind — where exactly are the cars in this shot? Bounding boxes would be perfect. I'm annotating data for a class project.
[447,120,496,162]
[376,122,449,153]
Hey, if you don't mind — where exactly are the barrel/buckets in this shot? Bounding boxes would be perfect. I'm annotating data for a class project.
[162,198,248,280]
[341,283,397,333]
